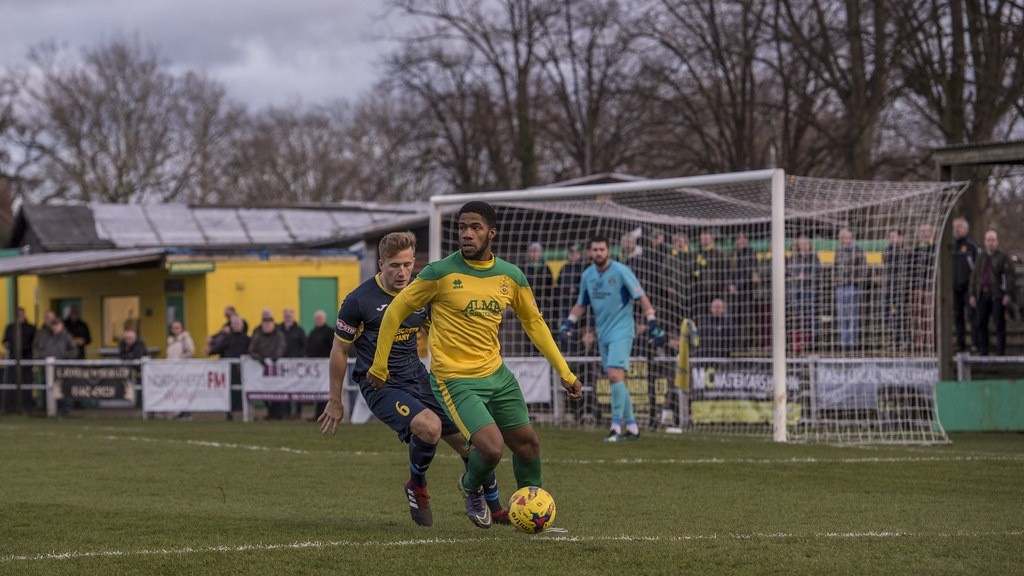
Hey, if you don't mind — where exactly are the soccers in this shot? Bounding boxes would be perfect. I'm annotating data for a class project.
[508,485,556,534]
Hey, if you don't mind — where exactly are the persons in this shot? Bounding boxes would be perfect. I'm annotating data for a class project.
[557,235,667,442]
[316,232,509,526]
[165,319,196,419]
[306,311,334,423]
[119,329,148,409]
[33,303,92,413]
[4,307,36,412]
[366,202,582,528]
[201,305,306,420]
[511,217,1015,430]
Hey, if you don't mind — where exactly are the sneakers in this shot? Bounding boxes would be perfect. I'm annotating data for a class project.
[405,479,434,526]
[604,429,624,441]
[491,508,512,525]
[457,472,493,529]
[623,431,642,440]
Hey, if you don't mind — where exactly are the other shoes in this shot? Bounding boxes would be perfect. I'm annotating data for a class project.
[662,410,673,425]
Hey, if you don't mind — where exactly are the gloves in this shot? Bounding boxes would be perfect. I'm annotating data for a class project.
[648,320,667,344]
[558,321,575,343]
